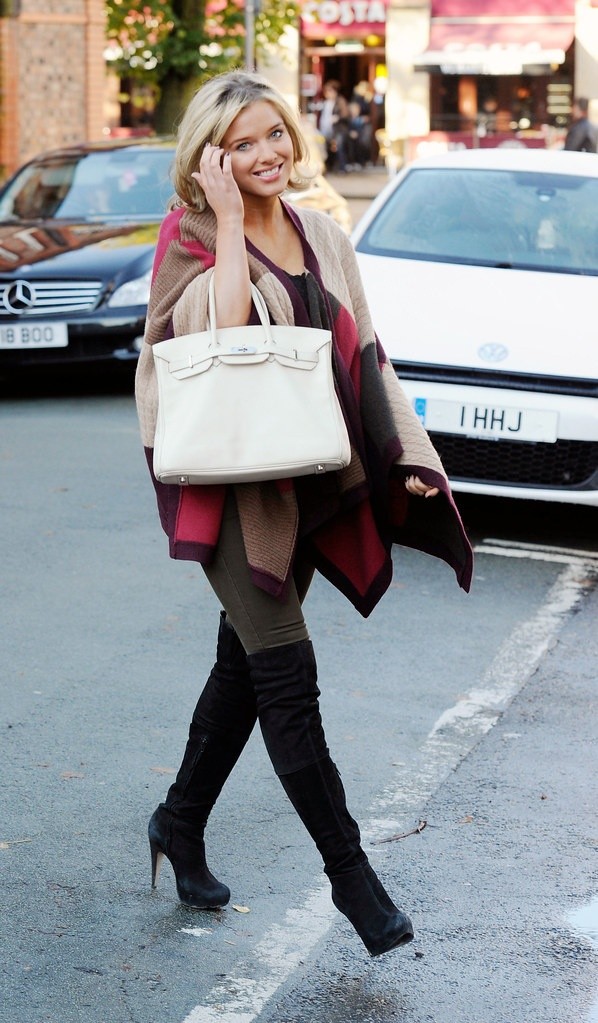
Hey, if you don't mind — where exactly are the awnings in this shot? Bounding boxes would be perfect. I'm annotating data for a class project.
[415,22,575,76]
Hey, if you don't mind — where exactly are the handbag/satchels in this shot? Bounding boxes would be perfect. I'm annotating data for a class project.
[152,270,351,485]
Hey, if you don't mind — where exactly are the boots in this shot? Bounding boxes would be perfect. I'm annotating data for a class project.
[246,640,413,957]
[148,610,258,910]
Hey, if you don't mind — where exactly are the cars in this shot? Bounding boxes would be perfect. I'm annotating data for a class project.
[0,136,181,399]
[346,148,598,532]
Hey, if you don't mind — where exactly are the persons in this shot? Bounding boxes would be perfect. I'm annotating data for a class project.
[512,98,533,132]
[564,99,598,154]
[510,192,589,262]
[135,69,474,958]
[476,95,498,137]
[318,81,375,171]
[74,170,116,214]
